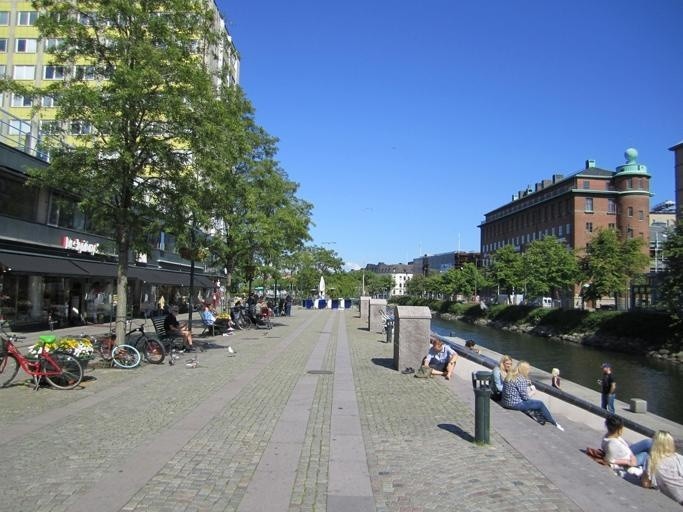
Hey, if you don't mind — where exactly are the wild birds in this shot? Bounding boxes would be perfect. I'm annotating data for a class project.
[227,345,237,357]
[168,348,199,368]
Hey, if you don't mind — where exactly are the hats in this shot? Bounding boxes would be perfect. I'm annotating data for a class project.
[600,362,614,369]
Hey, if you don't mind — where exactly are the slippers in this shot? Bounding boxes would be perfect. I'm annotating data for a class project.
[402,367,415,374]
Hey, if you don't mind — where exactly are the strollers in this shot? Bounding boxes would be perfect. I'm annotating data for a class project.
[378,309,395,335]
[255,308,273,329]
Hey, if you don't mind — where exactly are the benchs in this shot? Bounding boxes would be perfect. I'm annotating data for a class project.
[148,303,223,354]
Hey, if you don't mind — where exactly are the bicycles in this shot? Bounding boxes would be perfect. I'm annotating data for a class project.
[230,306,252,329]
[2,321,83,389]
[83,316,167,368]
[47,308,56,331]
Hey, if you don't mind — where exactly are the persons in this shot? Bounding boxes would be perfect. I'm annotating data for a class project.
[551,368,561,390]
[490,355,512,402]
[641,429,683,505]
[199,289,294,336]
[601,413,652,471]
[164,306,195,351]
[596,363,616,414]
[501,359,565,432]
[465,339,479,354]
[421,339,458,380]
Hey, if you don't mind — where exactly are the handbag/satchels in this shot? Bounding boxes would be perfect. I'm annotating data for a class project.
[490,375,502,401]
[587,446,608,465]
[415,367,432,378]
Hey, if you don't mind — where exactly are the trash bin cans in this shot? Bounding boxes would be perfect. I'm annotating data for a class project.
[303,298,352,309]
